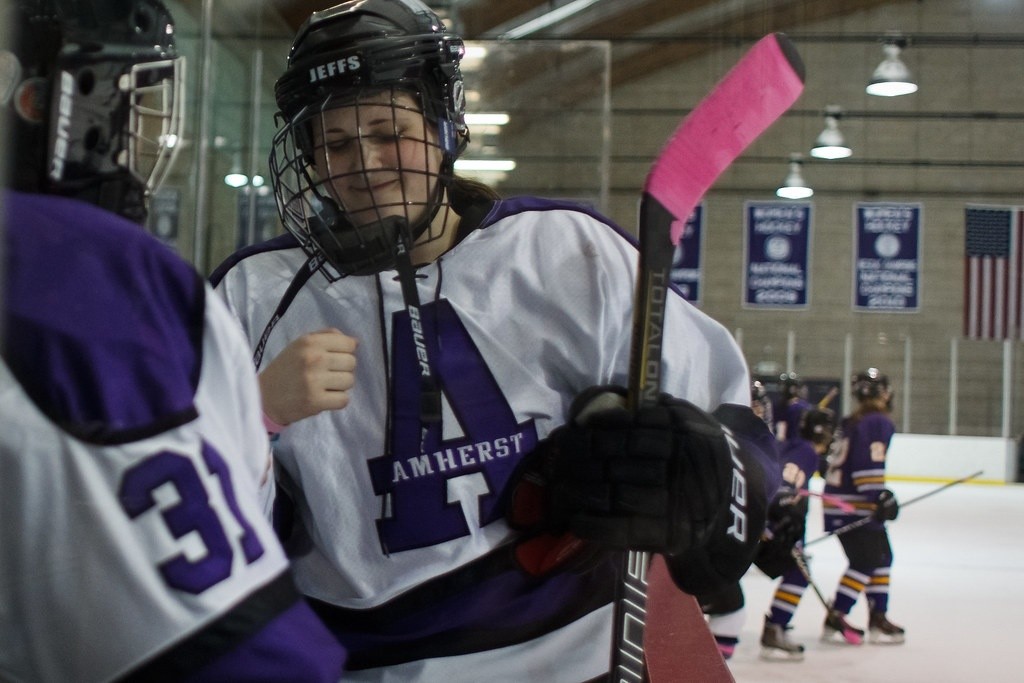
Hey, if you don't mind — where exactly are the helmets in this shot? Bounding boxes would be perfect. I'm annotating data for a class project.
[275,1,474,276]
[853,367,889,403]
[802,408,836,443]
[0,1,178,224]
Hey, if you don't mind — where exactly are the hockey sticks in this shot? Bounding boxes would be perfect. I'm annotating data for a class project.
[760,526,866,647]
[800,466,990,549]
[713,633,737,662]
[606,26,812,683]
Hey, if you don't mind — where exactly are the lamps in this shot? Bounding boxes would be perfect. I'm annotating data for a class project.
[809,117,853,159]
[776,162,813,199]
[865,44,918,96]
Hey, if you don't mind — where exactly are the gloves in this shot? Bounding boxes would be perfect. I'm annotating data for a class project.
[876,489,900,523]
[783,532,803,557]
[509,386,766,595]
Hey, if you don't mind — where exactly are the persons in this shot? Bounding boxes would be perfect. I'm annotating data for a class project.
[773,383,809,444]
[752,406,834,662]
[1,2,347,682]
[207,1,780,683]
[818,365,905,645]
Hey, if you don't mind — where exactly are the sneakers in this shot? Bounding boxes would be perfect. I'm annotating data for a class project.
[821,613,865,643]
[759,614,805,661]
[869,612,905,646]
